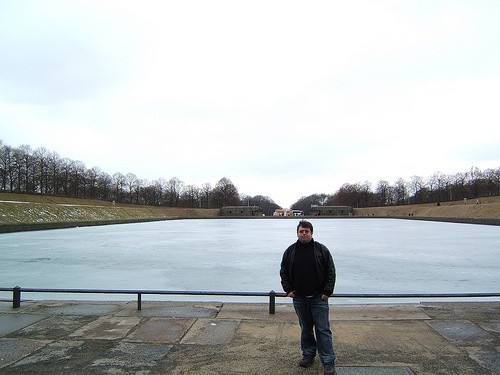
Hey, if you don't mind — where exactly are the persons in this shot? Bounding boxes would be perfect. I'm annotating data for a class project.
[280,219,338,375]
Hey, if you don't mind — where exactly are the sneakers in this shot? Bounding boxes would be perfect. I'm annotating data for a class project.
[299,356,315,366]
[324,364,335,375]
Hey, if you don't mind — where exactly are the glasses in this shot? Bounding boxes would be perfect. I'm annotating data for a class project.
[299,230,309,233]
